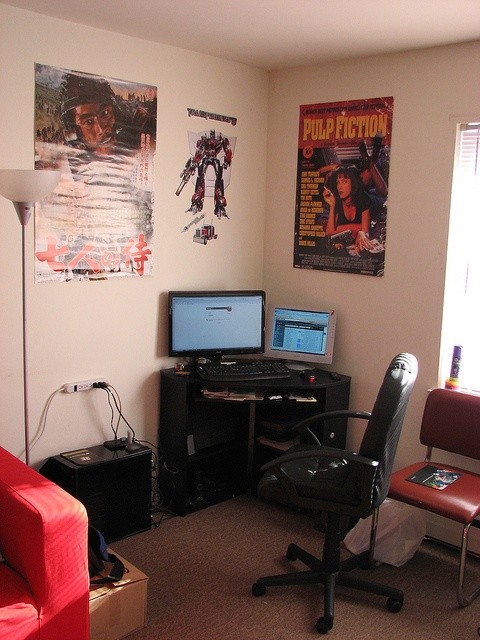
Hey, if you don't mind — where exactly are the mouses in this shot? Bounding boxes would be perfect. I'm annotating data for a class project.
[309,375,316,384]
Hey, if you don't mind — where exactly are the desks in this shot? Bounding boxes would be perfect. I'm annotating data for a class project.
[158,358,352,518]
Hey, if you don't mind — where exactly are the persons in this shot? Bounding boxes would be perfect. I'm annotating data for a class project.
[323,133,388,251]
[58,74,156,171]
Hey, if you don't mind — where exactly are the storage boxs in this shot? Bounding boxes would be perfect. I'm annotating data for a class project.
[90,545,149,639]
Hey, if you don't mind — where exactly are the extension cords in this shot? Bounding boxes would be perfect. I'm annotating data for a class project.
[64,378,109,393]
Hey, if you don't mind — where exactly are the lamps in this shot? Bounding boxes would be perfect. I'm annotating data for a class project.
[0,171,59,470]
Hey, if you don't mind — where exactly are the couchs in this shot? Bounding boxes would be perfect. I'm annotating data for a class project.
[2,445,89,639]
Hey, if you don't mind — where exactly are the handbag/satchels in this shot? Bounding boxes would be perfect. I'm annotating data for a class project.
[88,525,108,579]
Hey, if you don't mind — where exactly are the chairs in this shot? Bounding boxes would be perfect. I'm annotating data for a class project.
[367,389,480,608]
[248,354,418,632]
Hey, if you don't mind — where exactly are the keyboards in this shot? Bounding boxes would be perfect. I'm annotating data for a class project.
[194,359,292,381]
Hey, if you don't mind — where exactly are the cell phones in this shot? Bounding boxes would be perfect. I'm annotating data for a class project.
[329,372,341,381]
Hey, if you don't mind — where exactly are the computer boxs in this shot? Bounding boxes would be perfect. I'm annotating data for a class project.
[51,446,152,545]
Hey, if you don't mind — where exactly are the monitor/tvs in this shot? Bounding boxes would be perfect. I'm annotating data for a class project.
[263,304,337,371]
[168,290,266,359]
